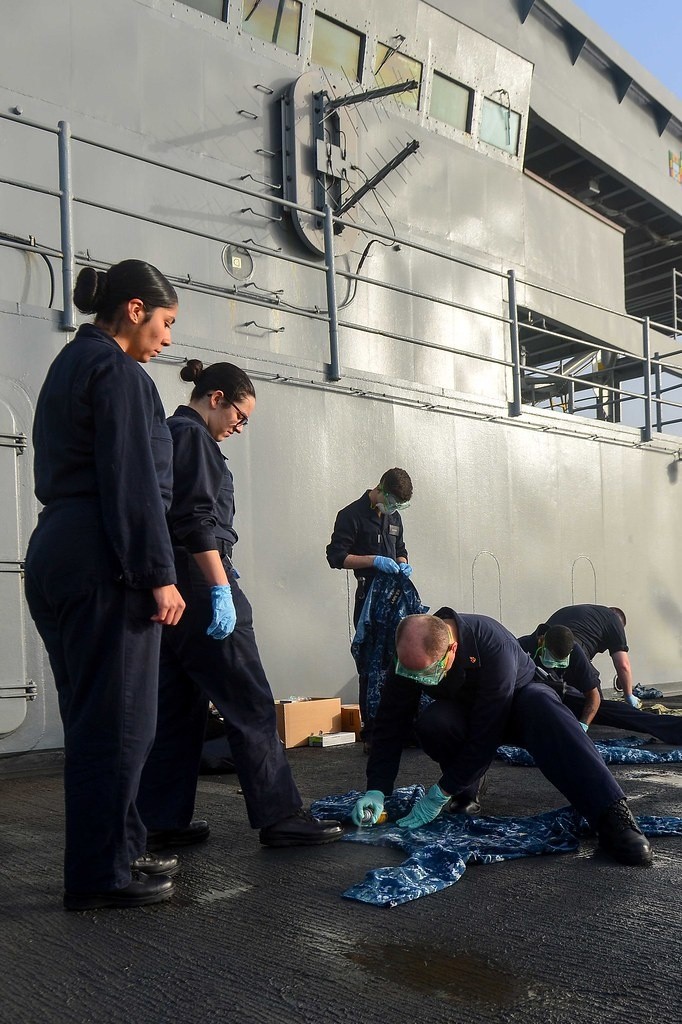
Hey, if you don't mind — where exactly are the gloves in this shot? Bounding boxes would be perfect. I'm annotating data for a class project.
[401,563,411,576]
[396,784,451,829]
[373,556,400,575]
[626,694,639,707]
[352,790,384,827]
[579,722,589,733]
[207,585,237,640]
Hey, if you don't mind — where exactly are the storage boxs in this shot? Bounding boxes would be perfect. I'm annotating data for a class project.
[277,696,361,748]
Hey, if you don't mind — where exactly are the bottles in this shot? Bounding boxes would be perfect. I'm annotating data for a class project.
[288,694,312,701]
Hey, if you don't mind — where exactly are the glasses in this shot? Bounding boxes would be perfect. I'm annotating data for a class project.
[540,644,571,668]
[393,650,449,685]
[207,392,249,427]
[383,489,412,510]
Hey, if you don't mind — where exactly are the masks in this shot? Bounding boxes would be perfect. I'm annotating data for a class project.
[376,503,396,515]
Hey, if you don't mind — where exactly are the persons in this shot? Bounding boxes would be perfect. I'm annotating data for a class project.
[326,467,412,754]
[350,607,653,866]
[515,623,601,734]
[142,359,344,848]
[25,260,187,909]
[543,604,645,710]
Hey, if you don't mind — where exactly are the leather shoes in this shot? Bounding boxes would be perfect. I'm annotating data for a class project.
[448,773,489,817]
[146,819,210,849]
[61,871,177,909]
[595,799,654,863]
[259,807,344,847]
[130,850,182,877]
